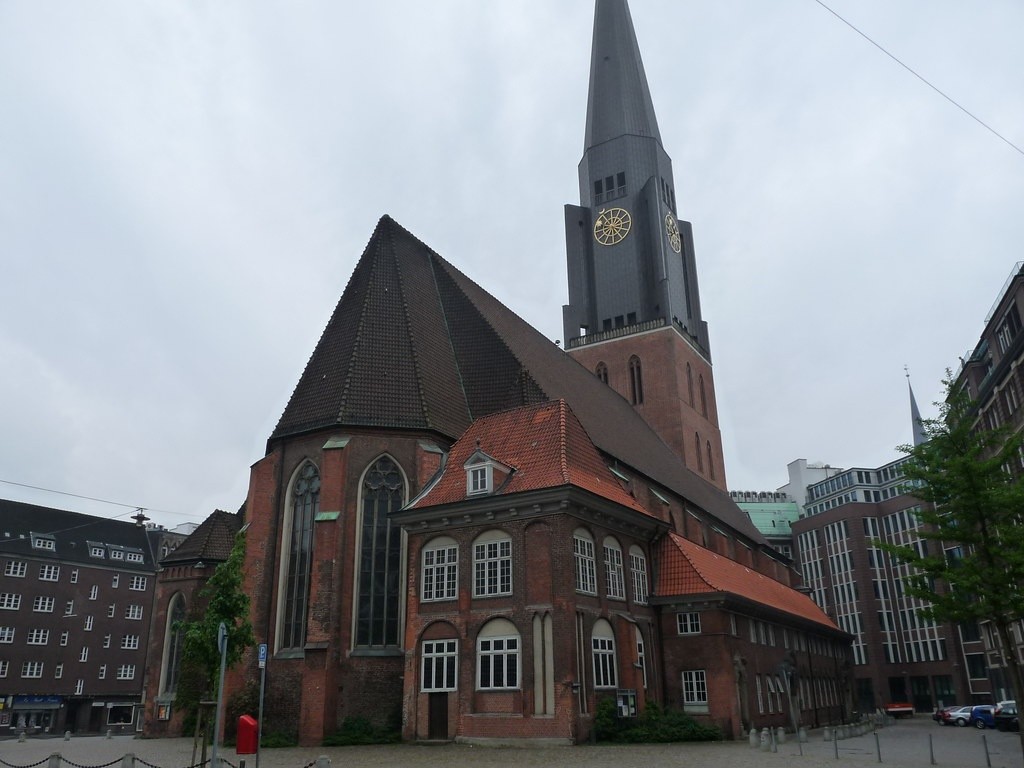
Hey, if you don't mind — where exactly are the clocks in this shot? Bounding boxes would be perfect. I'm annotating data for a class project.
[594,207,632,246]
[664,211,682,253]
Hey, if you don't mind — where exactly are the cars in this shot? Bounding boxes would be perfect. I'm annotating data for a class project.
[970,705,995,729]
[932,706,972,728]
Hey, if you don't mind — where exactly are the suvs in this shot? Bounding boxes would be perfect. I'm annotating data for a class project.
[993,702,1020,732]
